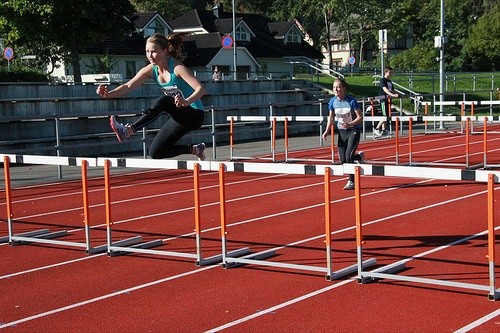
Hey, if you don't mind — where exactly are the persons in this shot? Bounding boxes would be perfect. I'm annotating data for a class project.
[96,32,207,162]
[321,77,364,190]
[362,97,379,114]
[373,67,404,139]
[212,70,221,81]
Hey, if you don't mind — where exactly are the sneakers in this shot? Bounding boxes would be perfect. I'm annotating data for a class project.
[357,151,364,164]
[343,180,355,189]
[109,114,130,142]
[196,143,205,161]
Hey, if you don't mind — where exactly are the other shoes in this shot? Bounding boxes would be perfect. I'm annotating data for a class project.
[374,129,382,136]
[381,130,388,134]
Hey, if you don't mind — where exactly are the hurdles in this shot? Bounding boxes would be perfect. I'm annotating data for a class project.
[0,154,500,300]
[480,100,500,132]
[227,115,500,169]
[420,101,478,134]
